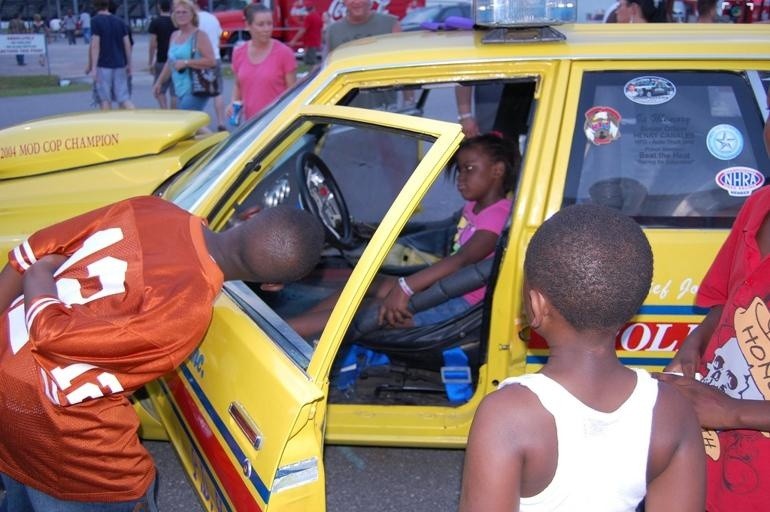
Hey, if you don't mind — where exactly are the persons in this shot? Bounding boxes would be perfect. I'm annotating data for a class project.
[653,84,770,512]
[287,4,323,70]
[615,0,770,25]
[285,134,516,342]
[225,4,297,125]
[453,84,504,137]
[86,4,134,110]
[1,194,324,512]
[10,8,91,66]
[458,205,706,512]
[148,0,227,130]
[323,0,414,108]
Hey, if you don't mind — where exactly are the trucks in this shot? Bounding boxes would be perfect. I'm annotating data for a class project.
[200,0,429,64]
[399,1,660,29]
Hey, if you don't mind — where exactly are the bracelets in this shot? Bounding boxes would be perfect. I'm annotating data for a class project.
[397,276,415,298]
[457,114,471,119]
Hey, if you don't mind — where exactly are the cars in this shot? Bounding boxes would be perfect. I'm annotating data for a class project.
[0,0,770,510]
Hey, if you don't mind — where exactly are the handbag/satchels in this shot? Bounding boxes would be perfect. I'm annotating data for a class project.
[189,58,222,96]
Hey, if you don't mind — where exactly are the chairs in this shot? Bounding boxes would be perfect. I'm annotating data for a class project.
[352,297,485,370]
